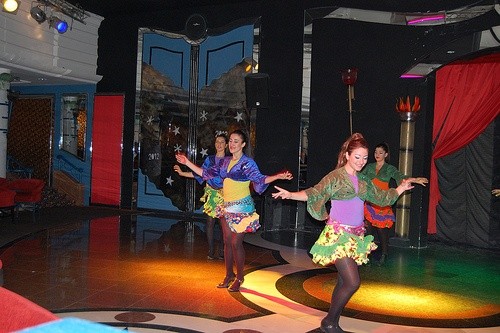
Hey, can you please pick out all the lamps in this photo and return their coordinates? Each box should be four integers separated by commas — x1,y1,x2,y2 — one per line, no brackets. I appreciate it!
241,60,251,72
2,0,68,34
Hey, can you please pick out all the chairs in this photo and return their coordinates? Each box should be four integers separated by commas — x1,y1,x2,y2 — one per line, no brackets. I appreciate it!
0,177,47,217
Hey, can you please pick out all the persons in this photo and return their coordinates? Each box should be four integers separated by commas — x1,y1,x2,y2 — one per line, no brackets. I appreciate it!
269,130,415,333
348,141,430,264
174,133,230,259
491,188,500,197
175,129,293,292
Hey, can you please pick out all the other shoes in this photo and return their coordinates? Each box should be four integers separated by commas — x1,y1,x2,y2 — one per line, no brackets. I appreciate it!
219,247,224,260
364,254,375,266
377,254,387,266
207,248,216,259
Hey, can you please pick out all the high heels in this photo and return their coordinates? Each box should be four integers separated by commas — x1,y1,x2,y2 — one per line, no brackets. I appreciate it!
228,278,244,292
320,317,344,333
217,272,236,288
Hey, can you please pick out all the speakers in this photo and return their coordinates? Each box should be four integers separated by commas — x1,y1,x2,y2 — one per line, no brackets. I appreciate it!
246,74,272,109
